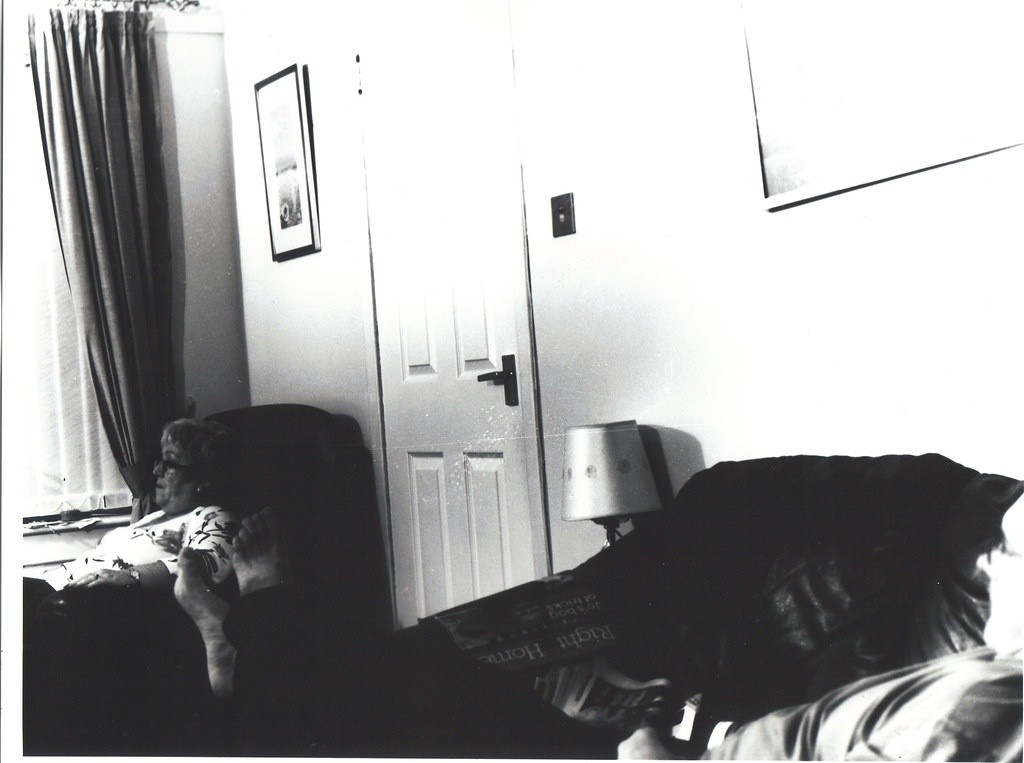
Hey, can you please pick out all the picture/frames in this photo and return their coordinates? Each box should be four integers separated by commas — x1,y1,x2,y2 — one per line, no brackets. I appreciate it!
252,62,323,264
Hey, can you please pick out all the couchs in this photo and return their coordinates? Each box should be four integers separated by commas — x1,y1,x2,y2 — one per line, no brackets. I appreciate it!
22,402,386,760
389,453,1022,762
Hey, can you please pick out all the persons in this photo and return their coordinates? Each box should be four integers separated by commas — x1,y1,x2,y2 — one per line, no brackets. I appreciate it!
24,419,237,625
175,485,1024,763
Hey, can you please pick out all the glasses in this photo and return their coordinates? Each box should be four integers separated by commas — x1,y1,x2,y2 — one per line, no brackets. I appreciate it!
987,544,1024,564
153,458,195,474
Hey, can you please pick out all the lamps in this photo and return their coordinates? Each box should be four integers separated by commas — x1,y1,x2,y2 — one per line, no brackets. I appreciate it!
561,420,664,554
